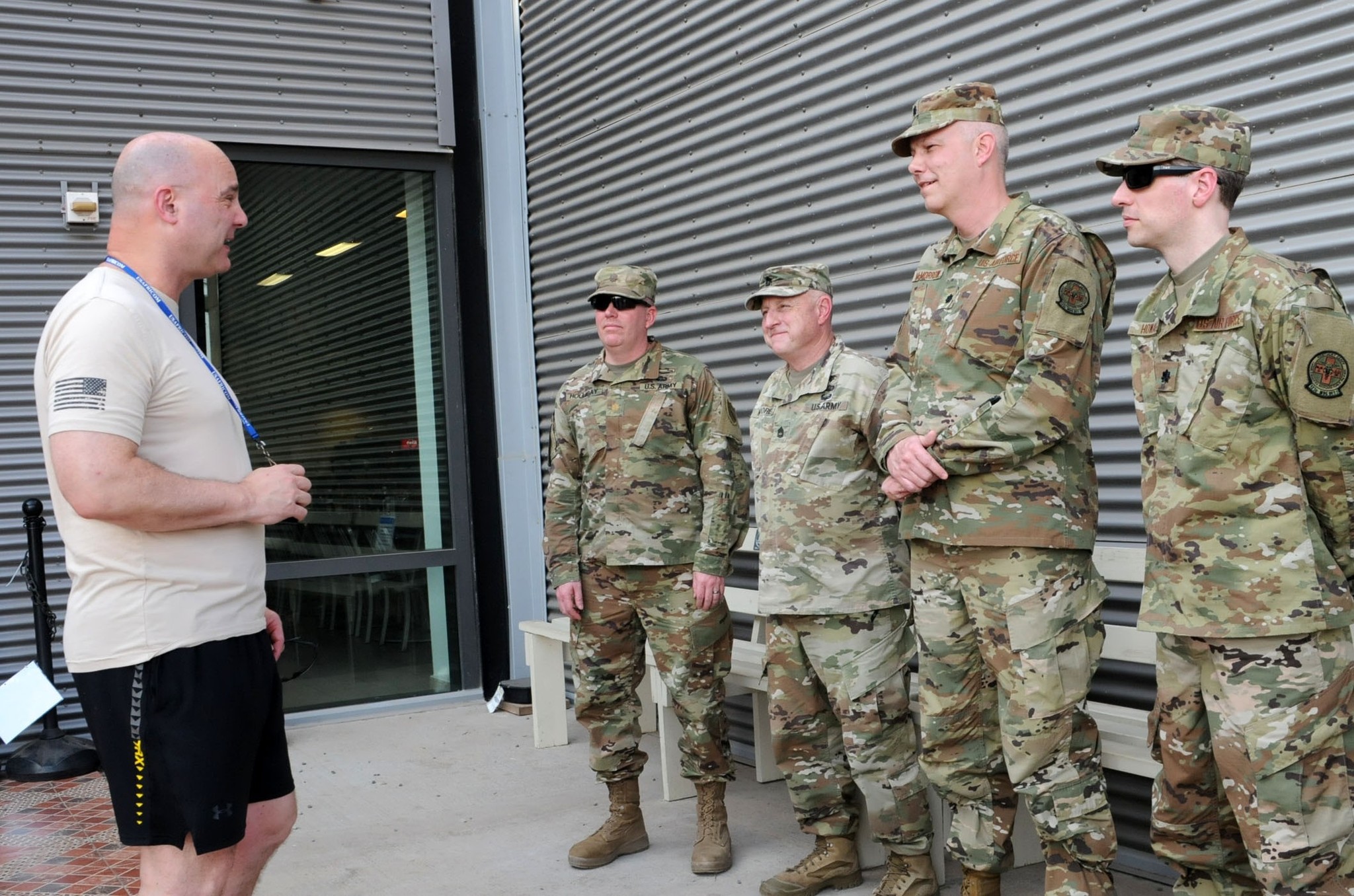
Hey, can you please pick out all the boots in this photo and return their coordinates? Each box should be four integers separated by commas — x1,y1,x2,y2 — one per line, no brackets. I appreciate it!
567,776,649,869
690,781,732,873
959,865,1001,896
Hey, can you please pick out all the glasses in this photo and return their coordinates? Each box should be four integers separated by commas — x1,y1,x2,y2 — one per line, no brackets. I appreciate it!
1122,165,1224,189
591,294,650,311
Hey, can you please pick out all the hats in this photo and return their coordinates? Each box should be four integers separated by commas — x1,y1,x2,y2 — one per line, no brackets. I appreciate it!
1095,103,1252,177
891,82,1005,157
587,265,658,302
745,263,834,310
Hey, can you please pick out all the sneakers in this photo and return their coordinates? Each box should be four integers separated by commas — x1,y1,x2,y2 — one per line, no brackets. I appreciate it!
759,835,862,896
873,853,940,896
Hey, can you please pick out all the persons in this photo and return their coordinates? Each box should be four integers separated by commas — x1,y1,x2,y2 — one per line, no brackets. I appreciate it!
34,132,311,896
544,264,748,874
864,82,1117,896
747,265,940,896
1097,106,1354,896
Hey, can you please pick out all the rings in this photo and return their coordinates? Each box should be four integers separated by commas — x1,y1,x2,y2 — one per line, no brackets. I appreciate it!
712,591,720,594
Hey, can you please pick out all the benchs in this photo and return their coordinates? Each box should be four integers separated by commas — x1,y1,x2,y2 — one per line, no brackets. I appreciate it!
519,524,788,802
907,543,1182,888
269,496,426,658
